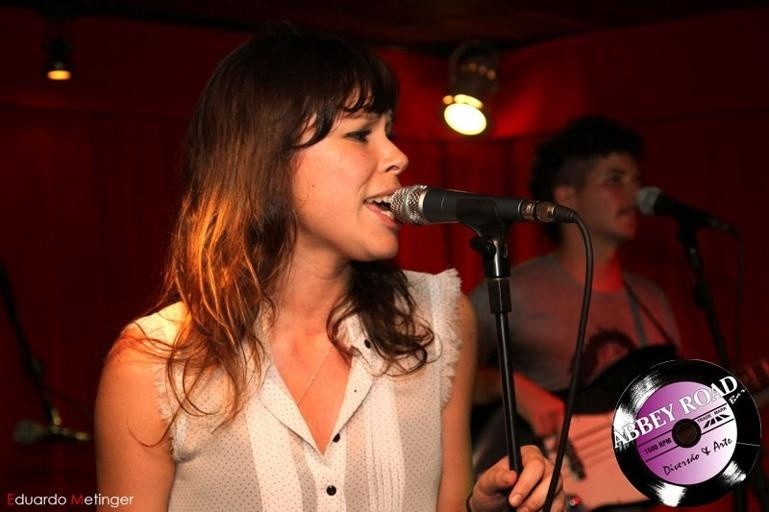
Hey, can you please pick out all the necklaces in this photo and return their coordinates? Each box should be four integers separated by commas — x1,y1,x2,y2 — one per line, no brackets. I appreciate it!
295,343,335,408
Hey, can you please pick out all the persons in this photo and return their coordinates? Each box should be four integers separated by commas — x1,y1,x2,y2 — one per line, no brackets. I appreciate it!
92,24,582,512
465,112,685,437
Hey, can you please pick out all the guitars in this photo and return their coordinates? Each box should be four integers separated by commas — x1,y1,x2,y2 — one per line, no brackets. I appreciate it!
471,341,768,511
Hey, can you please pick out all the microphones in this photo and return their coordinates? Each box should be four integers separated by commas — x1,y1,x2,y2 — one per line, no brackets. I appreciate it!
390,185,556,225
635,187,738,236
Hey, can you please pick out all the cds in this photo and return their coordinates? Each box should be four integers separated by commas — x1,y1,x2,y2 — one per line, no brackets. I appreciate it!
610,356,762,510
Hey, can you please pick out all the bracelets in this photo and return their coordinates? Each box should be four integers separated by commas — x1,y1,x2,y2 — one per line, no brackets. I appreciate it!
466,494,471,511
